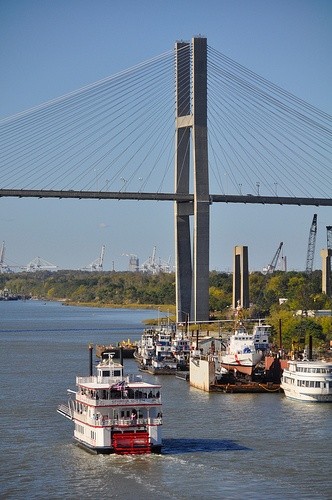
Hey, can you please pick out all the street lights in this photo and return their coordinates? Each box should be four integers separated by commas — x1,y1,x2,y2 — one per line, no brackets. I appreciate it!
238,183,243,196
139,178,143,193
178,309,189,340
255,181,261,196
273,182,278,196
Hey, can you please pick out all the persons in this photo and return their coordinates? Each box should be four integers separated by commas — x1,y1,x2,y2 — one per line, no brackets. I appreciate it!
129,411,137,422
91,387,161,400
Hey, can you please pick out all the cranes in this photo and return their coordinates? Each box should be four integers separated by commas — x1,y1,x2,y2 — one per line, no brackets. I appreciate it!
140,245,175,273
89,245,106,271
0,240,14,274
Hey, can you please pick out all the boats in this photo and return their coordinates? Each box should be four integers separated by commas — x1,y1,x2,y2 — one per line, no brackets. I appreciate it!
95,319,271,382
280,349,332,403
55,343,164,455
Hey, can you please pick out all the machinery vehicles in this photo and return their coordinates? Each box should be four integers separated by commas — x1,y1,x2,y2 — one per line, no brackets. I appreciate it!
301,214,318,318
255,241,284,306
